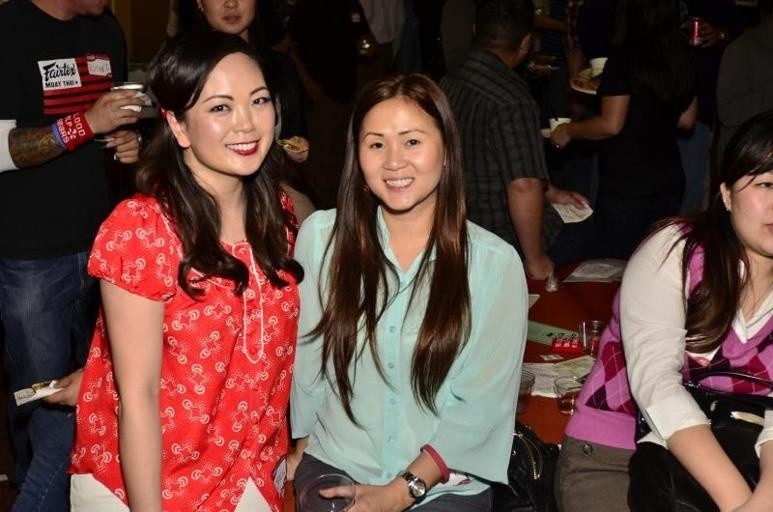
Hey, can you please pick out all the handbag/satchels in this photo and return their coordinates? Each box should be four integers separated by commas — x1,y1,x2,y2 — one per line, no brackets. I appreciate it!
491,419,560,511
626,383,773,512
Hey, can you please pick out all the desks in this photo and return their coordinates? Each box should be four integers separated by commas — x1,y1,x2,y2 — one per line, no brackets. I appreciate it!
512,259,623,445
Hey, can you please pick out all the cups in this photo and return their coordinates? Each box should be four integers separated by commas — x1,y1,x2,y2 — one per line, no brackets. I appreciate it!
549,117,572,134
579,318,604,353
589,56,606,74
298,470,357,511
108,84,145,116
552,373,588,415
514,369,536,417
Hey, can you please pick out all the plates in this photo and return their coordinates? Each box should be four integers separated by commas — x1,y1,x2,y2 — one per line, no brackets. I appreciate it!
578,67,591,80
539,128,552,139
567,77,597,96
